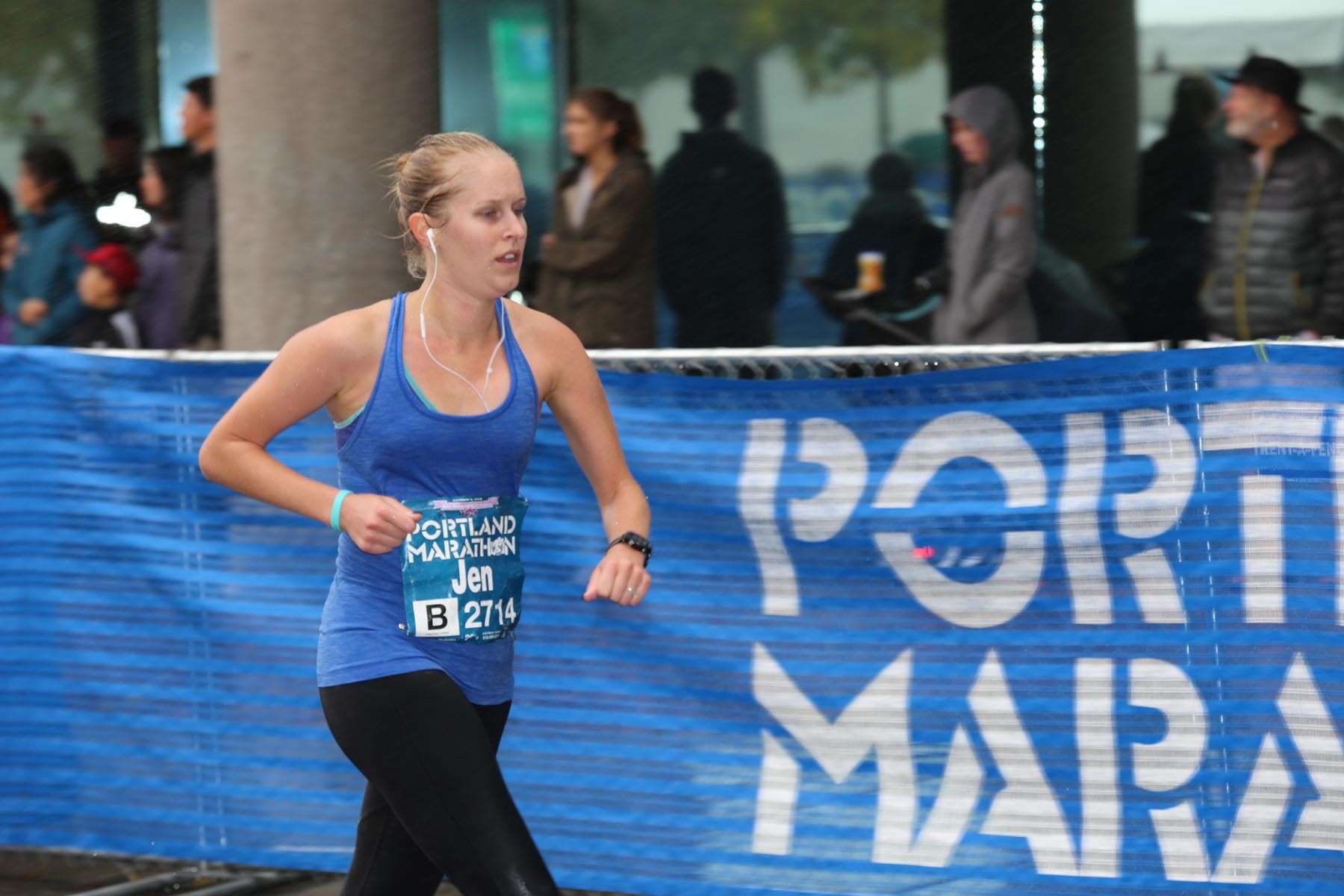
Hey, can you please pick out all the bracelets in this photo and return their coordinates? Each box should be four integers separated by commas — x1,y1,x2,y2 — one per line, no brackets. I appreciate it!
329,488,355,532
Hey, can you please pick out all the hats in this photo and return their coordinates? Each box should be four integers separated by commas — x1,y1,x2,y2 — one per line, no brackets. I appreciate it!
76,243,138,292
1212,53,1319,116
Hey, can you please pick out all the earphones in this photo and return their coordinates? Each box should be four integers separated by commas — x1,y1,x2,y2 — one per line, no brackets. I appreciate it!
426,227,438,253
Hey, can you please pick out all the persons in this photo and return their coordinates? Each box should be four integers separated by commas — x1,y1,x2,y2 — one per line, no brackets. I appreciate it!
501,50,1344,350
1,76,216,352
199,133,653,896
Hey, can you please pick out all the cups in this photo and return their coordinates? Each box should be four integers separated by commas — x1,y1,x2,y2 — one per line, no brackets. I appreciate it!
858,254,884,292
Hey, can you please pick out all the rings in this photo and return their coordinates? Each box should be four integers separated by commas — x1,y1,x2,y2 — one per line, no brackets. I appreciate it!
626,585,635,593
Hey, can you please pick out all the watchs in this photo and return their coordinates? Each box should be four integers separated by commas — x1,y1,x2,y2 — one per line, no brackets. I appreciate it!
602,531,651,567
